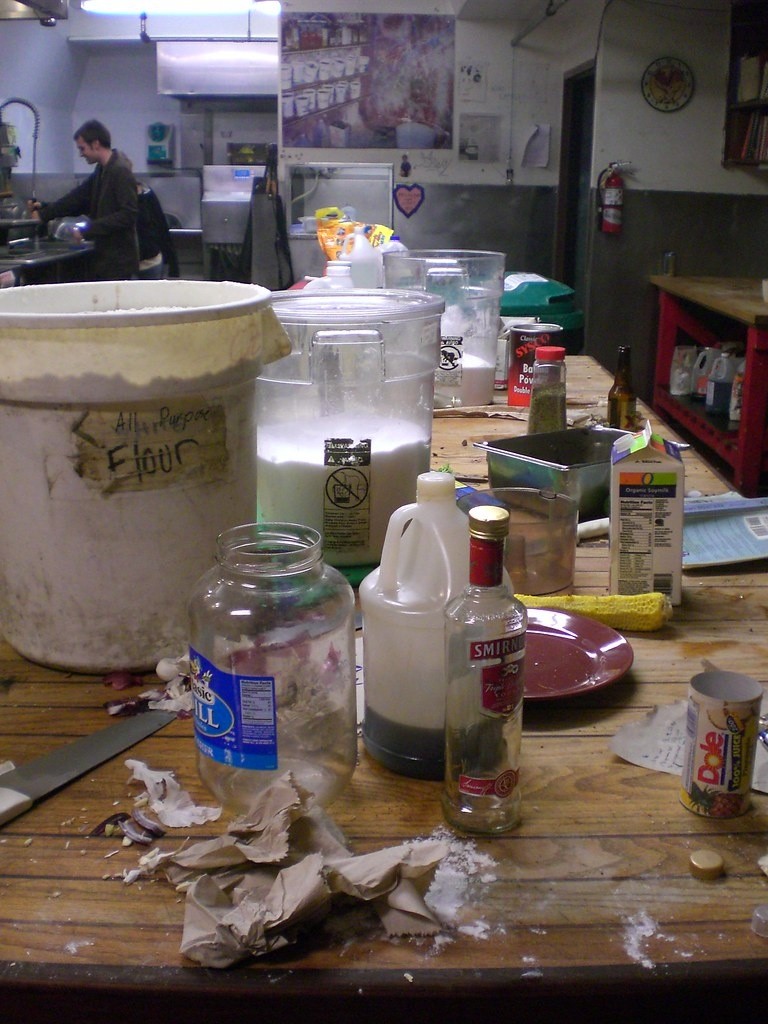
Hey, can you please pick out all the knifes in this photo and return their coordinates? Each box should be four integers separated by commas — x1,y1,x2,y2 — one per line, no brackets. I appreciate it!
0,710,177,826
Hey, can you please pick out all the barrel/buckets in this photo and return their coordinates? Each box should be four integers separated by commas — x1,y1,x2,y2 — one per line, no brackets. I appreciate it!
258,290,446,564
0,281,290,677
383,249,507,410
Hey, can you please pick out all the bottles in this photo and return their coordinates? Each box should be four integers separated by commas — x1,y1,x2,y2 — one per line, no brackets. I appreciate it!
608,346,637,433
360,471,512,782
189,523,357,815
528,346,568,435
706,352,736,418
692,347,714,406
444,505,528,834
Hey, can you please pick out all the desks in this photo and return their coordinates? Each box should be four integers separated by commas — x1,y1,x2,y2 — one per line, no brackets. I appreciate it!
0,355,768,990
649,272,768,494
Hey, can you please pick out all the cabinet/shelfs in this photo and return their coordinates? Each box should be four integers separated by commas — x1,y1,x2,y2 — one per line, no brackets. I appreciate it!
721,0,767,167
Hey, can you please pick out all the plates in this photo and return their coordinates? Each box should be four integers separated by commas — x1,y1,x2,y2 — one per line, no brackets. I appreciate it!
524,607,635,701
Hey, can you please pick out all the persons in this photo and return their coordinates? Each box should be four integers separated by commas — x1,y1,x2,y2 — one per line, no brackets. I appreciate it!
29,119,139,281
112,148,179,279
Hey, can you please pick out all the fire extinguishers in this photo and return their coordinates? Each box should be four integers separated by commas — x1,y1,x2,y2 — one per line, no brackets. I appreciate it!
596,159,633,235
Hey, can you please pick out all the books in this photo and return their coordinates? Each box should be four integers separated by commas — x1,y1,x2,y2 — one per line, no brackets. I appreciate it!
682,491,768,569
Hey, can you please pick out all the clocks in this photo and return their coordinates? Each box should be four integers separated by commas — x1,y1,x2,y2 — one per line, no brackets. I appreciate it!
641,57,693,111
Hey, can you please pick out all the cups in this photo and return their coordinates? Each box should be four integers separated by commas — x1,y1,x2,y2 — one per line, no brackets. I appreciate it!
509,323,563,406
458,487,578,599
677,673,765,818
